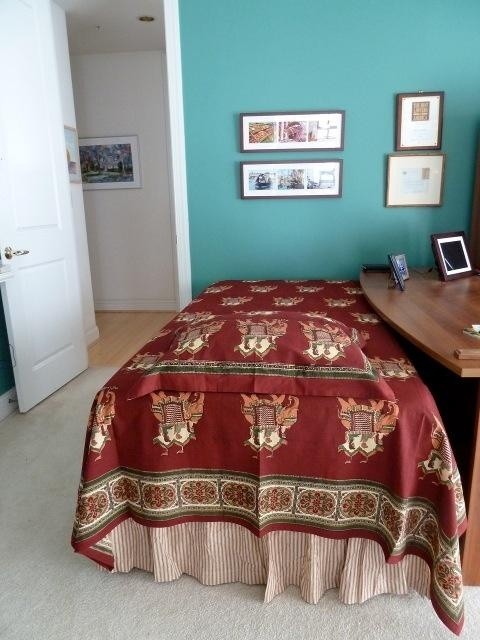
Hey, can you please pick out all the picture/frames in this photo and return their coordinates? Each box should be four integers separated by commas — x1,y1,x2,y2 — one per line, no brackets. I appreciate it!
388,230,478,291
386,90,447,209
239,109,344,201
61,124,143,193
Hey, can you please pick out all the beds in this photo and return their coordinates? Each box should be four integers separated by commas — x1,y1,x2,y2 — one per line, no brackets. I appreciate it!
97,278,453,596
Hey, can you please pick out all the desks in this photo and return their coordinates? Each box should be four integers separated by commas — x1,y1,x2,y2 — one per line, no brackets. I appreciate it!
356,266,478,593
